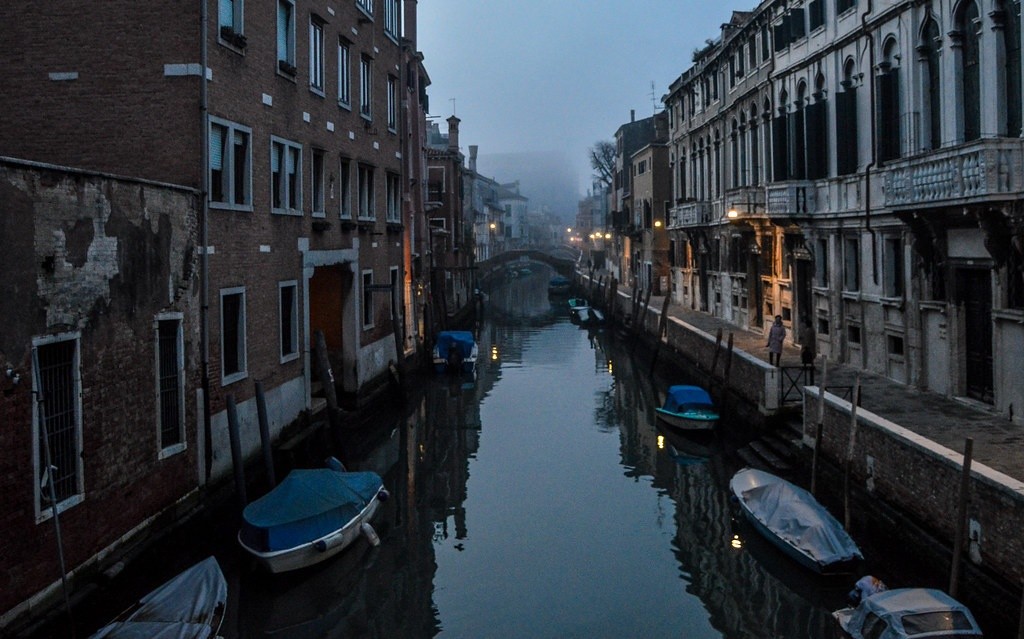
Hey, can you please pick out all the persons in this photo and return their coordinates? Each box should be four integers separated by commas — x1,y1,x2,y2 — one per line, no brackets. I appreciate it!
799,320,815,370
765,315,786,367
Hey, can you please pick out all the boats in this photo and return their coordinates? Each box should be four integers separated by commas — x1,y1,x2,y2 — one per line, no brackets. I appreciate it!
569,297,591,319
432,331,478,373
830,574,984,639
237,468,390,576
654,384,720,429
83,555,229,639
729,467,864,572
